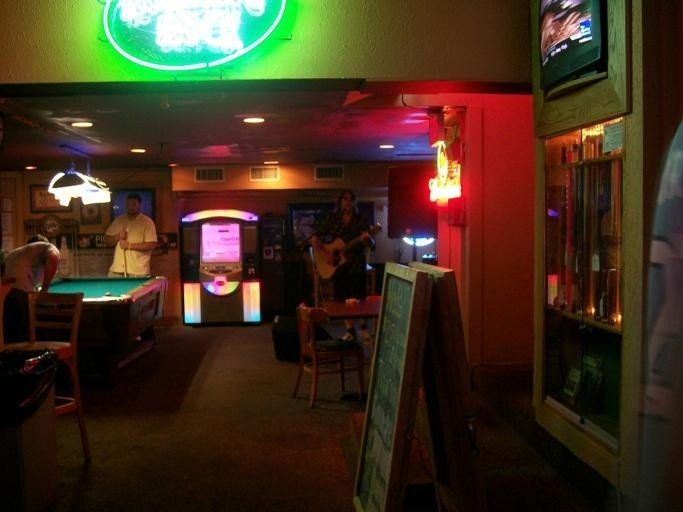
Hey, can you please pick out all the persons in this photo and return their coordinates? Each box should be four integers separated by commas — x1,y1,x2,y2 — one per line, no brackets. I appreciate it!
298,188,376,345
105,193,160,277
542,11,586,52
0,234,59,342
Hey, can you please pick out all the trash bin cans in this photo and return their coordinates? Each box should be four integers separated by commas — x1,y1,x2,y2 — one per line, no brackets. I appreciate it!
0,348,58,512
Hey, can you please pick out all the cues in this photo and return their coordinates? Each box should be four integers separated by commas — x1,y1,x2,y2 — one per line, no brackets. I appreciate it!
121,223,127,277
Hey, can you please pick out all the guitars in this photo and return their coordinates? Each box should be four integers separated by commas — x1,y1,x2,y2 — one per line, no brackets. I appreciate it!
316,222,382,280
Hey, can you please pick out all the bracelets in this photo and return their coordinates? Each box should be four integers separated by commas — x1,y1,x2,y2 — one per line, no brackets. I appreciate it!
128,243,133,250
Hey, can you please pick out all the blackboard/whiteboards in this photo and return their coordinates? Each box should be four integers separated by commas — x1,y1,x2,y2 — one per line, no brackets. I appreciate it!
352,263,428,512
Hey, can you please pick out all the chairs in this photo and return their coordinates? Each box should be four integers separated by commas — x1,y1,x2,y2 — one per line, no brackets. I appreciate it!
292,302,365,408
5,292,93,465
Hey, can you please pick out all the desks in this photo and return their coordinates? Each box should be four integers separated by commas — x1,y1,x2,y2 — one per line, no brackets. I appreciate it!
328,300,381,375
33,276,168,358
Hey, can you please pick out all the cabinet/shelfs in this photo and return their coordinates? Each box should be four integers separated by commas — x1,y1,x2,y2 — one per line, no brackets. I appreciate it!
532,115,643,486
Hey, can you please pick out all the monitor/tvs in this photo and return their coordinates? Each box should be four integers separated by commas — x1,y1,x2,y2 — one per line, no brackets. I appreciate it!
536,0,608,90
109,187,156,225
